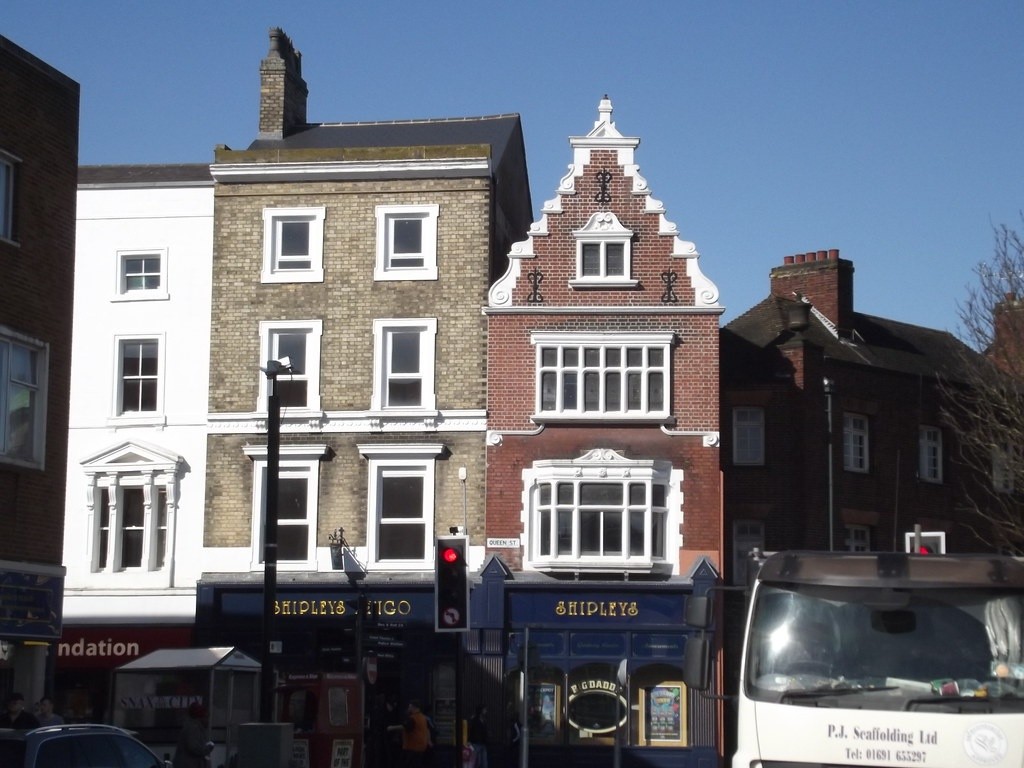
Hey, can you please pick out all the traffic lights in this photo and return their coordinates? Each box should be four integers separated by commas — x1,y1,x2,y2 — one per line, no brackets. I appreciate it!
436,537,471,636
905,531,946,558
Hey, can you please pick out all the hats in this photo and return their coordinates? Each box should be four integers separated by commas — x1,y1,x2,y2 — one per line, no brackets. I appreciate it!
190,703,208,718
6,693,24,701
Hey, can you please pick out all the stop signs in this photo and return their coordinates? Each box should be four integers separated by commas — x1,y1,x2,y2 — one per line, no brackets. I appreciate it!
365,655,376,683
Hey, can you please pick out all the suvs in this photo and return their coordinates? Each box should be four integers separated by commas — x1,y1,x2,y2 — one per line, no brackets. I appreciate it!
0,724,163,768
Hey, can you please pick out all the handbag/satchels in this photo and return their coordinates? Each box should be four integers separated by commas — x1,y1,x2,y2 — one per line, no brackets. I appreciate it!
462,745,476,768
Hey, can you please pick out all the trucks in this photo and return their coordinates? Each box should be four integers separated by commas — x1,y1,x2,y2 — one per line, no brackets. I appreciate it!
684,549,1024,768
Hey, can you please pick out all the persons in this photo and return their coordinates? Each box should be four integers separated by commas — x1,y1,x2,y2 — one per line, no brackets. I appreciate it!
468,704,489,768
172,703,216,768
374,698,438,768
33,696,66,727
0,692,41,729
